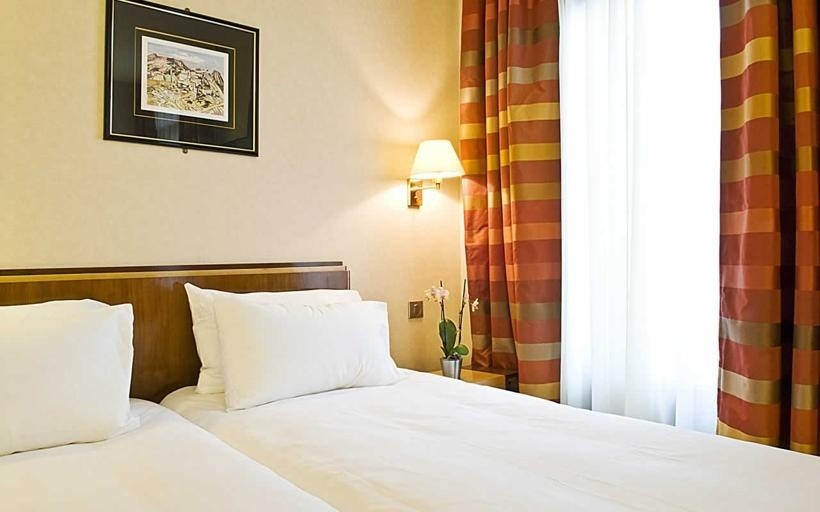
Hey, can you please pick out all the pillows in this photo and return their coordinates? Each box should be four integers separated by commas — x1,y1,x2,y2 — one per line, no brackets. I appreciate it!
0,299,136,456
212,293,400,412
181,282,363,393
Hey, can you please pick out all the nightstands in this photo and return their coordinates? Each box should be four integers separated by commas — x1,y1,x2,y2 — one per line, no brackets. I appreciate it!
430,368,506,389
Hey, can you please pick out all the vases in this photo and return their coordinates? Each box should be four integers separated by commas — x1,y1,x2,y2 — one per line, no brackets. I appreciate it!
441,357,461,381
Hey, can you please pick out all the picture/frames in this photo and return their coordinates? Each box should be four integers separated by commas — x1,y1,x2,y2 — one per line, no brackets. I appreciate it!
102,0,260,156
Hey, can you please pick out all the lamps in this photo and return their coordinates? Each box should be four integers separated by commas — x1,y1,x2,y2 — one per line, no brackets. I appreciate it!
405,139,468,208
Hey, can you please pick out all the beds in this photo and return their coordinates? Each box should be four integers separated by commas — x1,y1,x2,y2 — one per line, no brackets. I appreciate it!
0,398,342,512
161,362,819,512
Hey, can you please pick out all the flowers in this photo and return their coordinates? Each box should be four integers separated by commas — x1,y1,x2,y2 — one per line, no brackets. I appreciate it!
424,280,480,361
